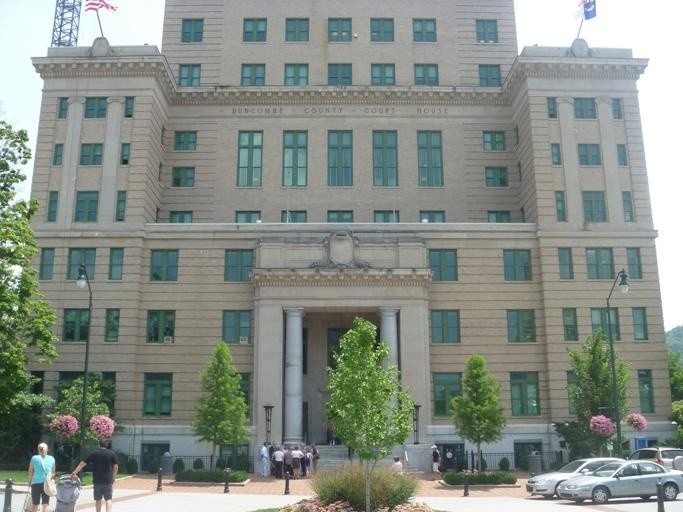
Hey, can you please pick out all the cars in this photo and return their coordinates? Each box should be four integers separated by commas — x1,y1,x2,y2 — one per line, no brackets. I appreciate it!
525,457,652,499
555,460,682,504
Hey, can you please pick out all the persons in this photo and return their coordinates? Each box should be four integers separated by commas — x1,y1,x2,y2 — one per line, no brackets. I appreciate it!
70,437,119,512
258,441,321,480
430,445,443,480
27,440,56,512
326,416,336,446
391,456,402,472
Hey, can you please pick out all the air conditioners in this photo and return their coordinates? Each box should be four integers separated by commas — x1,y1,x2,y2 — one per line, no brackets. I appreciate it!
164,336,172,344
239,336,248,345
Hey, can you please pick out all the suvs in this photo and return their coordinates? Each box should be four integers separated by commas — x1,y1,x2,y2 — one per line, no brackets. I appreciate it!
624,446,682,473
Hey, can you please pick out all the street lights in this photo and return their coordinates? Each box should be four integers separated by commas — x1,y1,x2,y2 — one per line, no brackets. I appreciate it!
261,402,274,447
76,260,93,480
605,267,629,456
412,401,422,444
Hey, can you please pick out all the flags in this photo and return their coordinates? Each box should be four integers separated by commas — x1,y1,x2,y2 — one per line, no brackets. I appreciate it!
576,0,597,21
84,0,119,14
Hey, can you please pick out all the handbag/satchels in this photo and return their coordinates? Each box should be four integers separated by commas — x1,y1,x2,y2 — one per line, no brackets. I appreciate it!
43,473,57,497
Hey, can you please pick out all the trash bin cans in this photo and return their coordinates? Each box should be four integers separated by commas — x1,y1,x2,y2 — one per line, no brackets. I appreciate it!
528,450,541,476
160,451,173,474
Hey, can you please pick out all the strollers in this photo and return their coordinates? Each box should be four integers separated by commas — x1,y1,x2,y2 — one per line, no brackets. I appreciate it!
20,482,43,512
54,473,81,512
